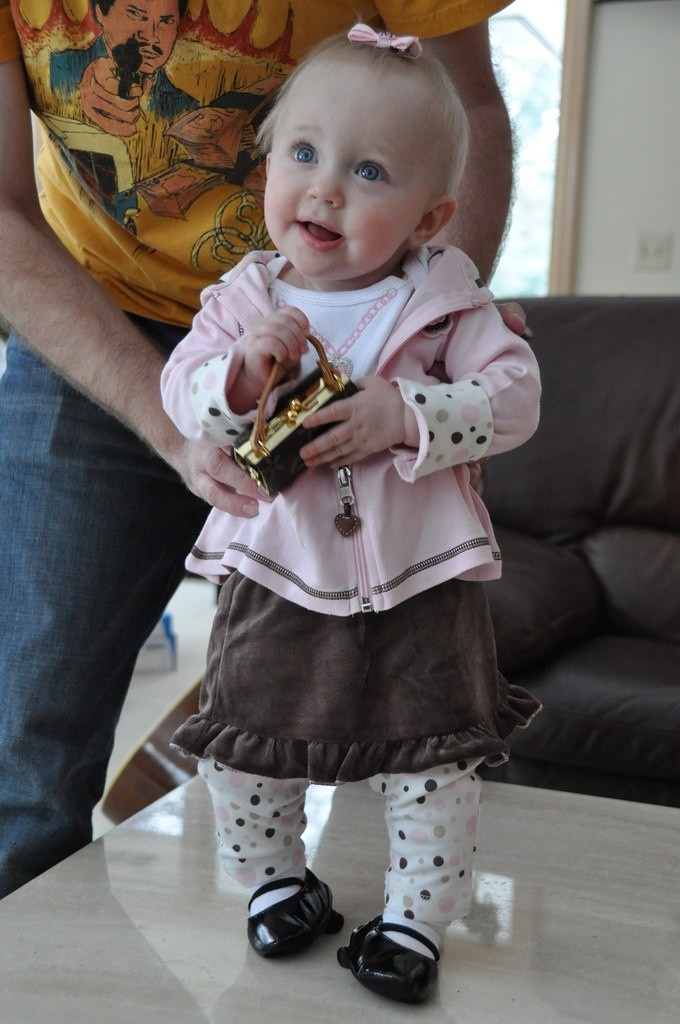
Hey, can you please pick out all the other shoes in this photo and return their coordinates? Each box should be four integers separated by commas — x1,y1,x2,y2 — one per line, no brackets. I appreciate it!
247,867,344,957
337,914,440,1004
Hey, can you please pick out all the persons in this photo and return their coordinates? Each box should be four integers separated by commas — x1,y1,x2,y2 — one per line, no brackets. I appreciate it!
160,22,544,1005
0,0,517,900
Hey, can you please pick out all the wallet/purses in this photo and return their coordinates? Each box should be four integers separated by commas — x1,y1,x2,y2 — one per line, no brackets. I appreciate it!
231,334,360,498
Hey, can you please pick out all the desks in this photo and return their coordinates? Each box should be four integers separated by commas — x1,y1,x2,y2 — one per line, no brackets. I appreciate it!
1,771,678,1024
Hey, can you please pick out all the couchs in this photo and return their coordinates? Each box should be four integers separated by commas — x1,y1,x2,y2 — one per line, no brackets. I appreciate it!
473,294,679,807
